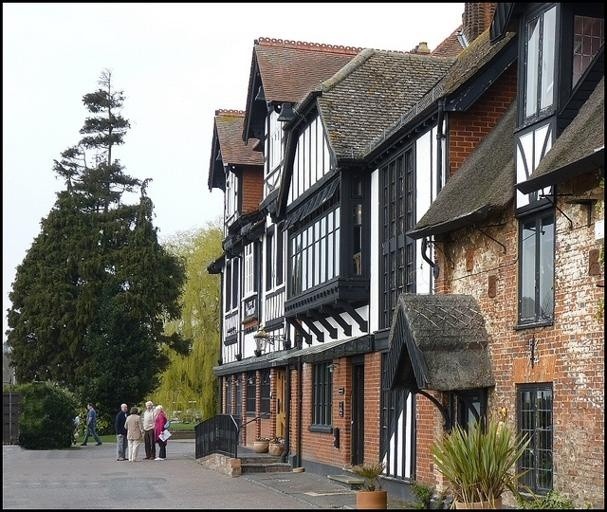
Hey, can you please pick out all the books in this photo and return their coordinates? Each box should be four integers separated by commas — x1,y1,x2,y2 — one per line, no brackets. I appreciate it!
158,429,172,442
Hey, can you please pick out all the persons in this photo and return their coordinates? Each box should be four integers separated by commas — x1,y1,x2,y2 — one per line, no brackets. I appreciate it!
141,401,160,461
122,407,144,462
78,403,103,446
152,405,169,461
113,403,129,461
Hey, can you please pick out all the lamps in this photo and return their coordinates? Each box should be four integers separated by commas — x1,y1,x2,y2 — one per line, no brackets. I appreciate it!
225,374,272,386
328,362,340,372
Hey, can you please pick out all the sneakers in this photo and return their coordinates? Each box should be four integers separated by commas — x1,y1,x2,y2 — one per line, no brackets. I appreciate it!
116,457,128,461
95,442,102,446
144,457,167,460
80,443,88,446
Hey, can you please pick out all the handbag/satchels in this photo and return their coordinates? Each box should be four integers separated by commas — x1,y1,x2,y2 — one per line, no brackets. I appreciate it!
159,420,173,442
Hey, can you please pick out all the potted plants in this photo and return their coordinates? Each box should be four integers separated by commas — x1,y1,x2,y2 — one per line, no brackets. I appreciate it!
268,436,284,456
253,435,270,452
409,482,447,510
427,416,541,509
352,463,387,509
